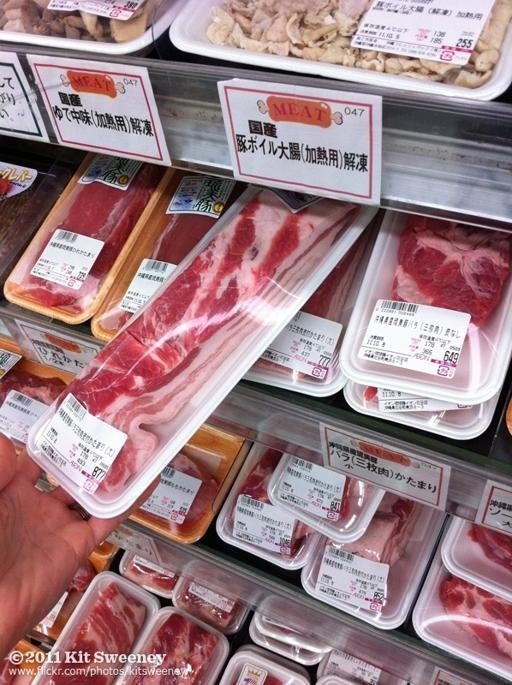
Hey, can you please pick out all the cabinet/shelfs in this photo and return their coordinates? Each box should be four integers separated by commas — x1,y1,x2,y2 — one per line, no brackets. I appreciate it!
0,1,512,685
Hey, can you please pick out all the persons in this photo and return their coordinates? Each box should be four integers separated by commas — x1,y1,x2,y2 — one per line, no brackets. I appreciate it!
0,436,160,661
